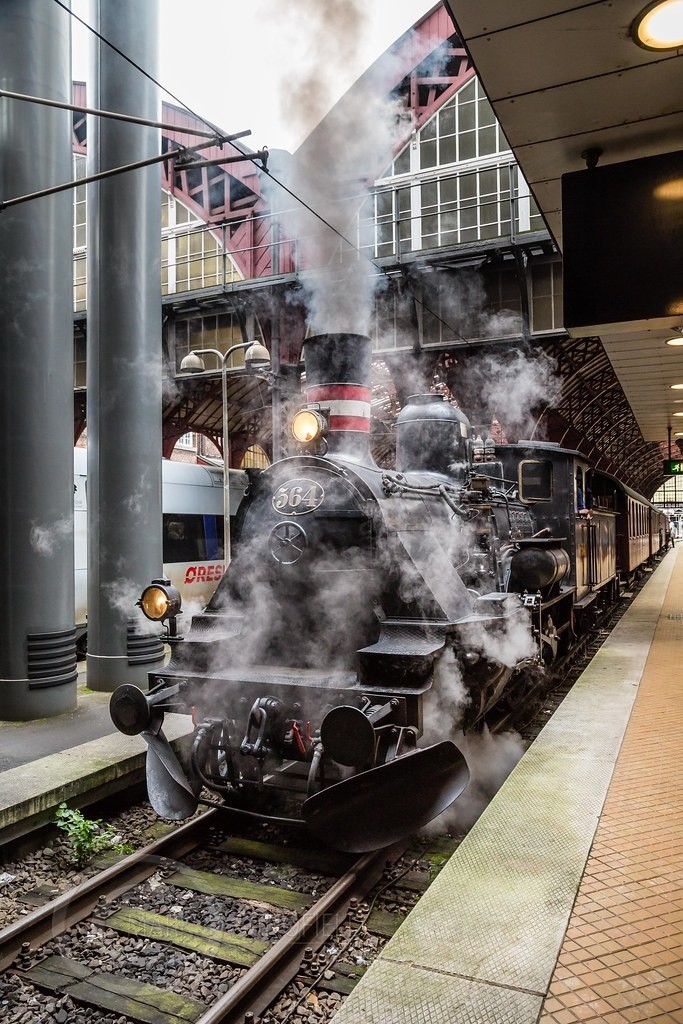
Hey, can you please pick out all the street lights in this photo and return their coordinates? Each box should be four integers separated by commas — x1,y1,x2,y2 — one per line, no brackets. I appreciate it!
177,337,278,574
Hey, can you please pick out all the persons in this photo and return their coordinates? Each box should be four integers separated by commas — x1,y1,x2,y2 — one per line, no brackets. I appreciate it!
659,522,676,551
577,474,594,520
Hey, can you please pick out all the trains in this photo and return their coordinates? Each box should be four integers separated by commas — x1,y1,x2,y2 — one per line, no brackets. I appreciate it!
64,447,275,648
102,334,674,858
